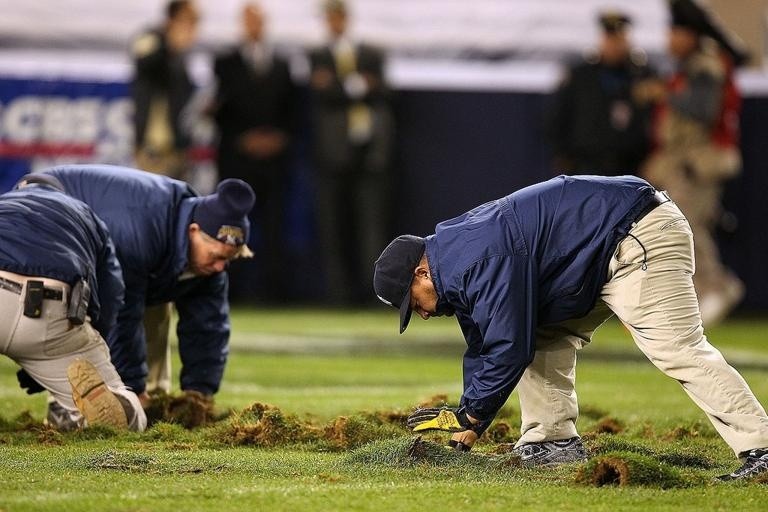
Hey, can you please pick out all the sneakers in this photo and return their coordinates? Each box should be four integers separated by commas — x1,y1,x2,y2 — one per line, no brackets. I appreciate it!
43,357,130,436
713,448,768,483
511,438,588,467
698,274,744,327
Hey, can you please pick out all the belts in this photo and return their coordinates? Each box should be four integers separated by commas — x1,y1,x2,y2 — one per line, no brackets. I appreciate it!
617,189,671,243
0,278,72,302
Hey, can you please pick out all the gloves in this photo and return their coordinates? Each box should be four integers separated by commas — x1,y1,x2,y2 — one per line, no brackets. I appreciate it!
408,405,474,432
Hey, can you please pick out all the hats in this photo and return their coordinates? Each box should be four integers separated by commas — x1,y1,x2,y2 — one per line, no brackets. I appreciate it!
191,177,257,247
372,232,428,335
597,11,632,32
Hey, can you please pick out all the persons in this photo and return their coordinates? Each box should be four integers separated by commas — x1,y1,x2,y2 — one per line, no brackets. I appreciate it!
214,1,311,303
634,0,751,326
0,174,148,434
299,0,391,308
374,174,768,481
131,0,219,186
542,12,665,183
13,165,255,426
